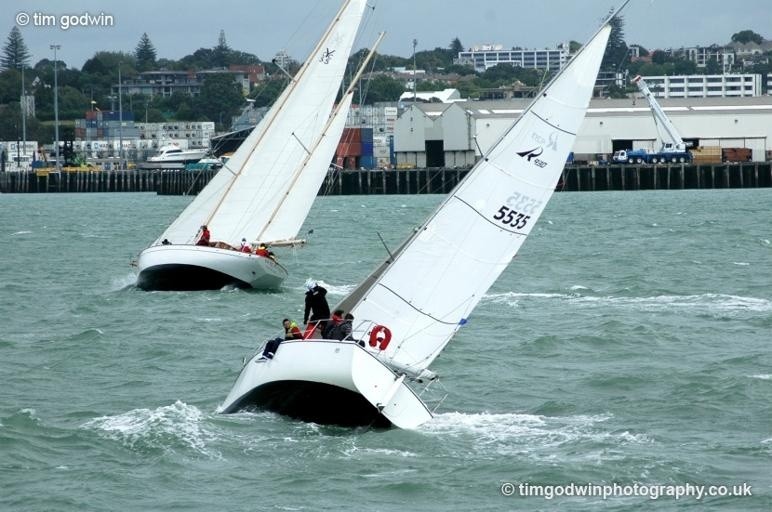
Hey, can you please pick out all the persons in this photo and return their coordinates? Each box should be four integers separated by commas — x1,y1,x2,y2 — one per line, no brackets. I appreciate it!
256,243,270,258
196,225,210,246
161,239,171,245
320,310,344,339
327,312,365,348
238,238,253,253
255,319,304,363
304,281,330,327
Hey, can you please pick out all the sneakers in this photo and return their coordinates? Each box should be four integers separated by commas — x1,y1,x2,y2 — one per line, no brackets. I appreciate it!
256,358,266,362
263,354,273,360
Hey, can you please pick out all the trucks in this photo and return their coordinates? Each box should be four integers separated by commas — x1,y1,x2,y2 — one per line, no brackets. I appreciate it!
614,148,647,164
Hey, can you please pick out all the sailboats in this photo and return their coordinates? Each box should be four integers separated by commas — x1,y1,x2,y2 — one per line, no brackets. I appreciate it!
132,1,388,290
216,2,628,430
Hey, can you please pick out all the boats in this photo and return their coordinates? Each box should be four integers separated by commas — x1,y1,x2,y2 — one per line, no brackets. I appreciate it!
147,148,203,164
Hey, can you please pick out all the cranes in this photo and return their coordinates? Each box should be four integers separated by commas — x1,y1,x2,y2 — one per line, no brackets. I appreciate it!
631,74,687,165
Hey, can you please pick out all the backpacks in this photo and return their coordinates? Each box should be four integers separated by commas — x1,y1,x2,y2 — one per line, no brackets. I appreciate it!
321,320,347,339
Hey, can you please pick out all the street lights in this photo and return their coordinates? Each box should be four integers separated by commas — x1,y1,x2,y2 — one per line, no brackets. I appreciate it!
50,45,61,169
